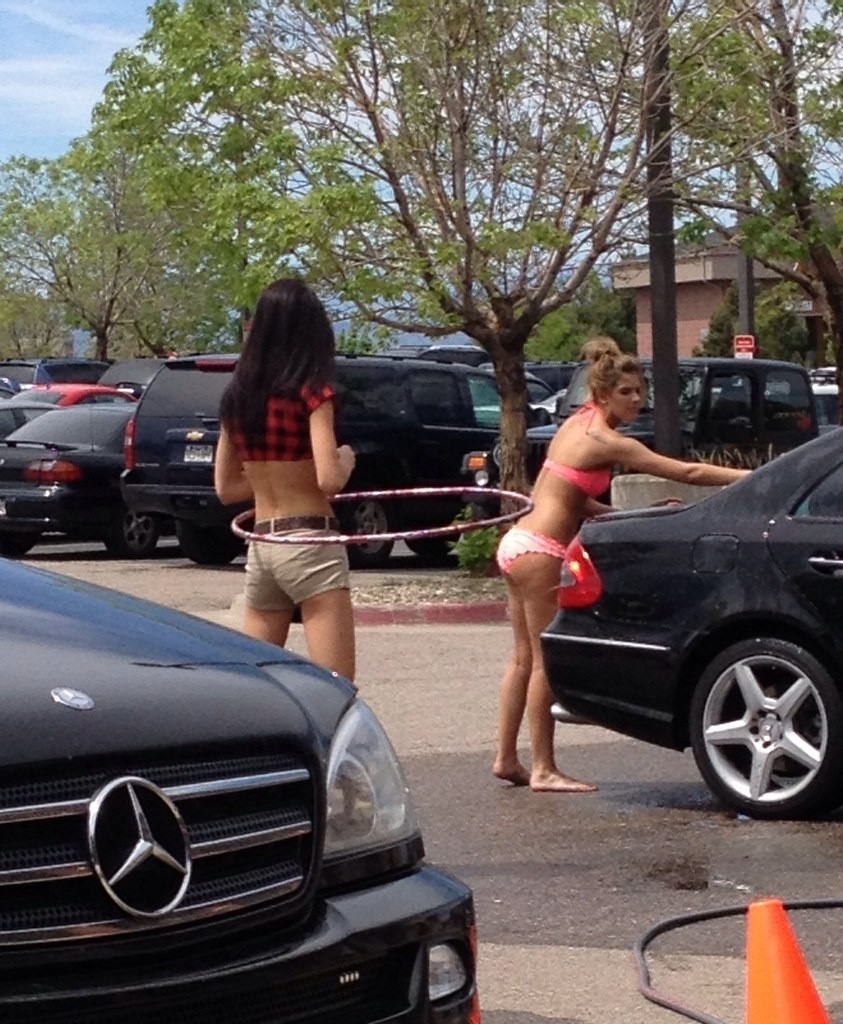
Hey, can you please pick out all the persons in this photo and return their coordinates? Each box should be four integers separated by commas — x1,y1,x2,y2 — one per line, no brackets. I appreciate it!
209,280,359,682
492,335,753,791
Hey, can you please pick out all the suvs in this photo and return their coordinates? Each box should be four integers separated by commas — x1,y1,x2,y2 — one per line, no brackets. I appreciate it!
116,354,554,570
1,343,843,567
0,551,482,1023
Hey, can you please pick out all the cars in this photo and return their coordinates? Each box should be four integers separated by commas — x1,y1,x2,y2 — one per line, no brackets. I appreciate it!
533,423,842,821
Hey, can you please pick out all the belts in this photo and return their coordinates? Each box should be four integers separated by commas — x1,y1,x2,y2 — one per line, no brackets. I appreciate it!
254,517,343,537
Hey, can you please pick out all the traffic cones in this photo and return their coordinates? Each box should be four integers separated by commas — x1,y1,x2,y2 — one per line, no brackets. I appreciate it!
742,898,831,1024
